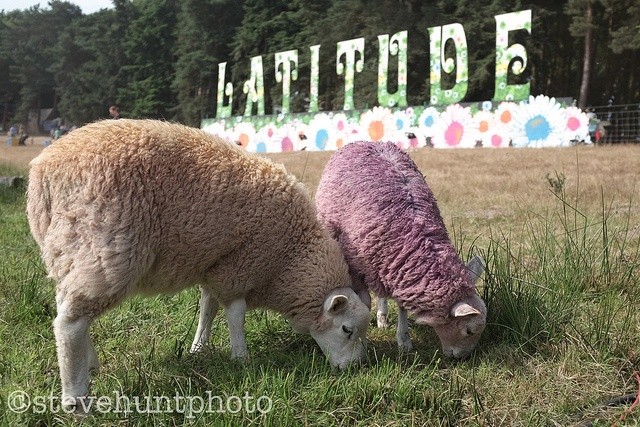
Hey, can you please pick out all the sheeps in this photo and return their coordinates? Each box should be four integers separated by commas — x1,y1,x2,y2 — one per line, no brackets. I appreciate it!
25,118,371,416
314,140,488,361
